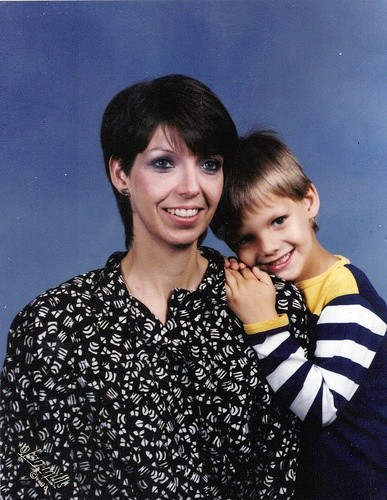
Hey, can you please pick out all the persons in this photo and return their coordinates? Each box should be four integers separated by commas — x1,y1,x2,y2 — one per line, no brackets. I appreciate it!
0,73,308,500
209,129,387,500
